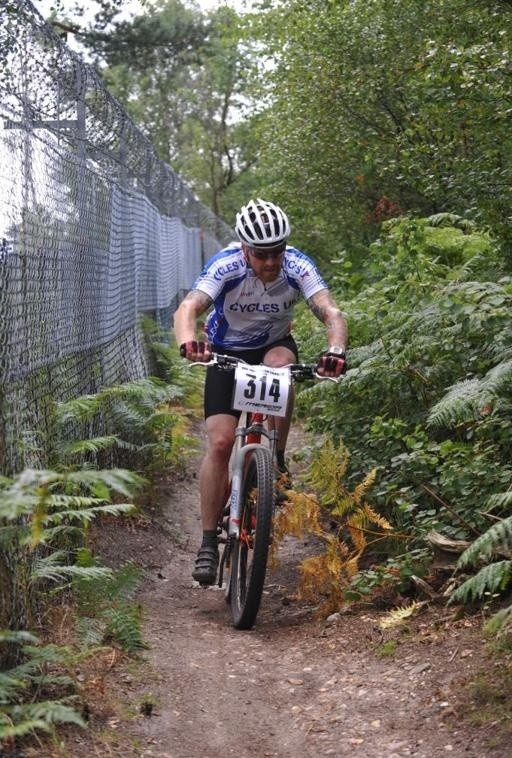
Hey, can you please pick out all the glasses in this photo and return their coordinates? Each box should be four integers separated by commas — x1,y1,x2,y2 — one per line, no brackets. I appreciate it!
249,243,286,260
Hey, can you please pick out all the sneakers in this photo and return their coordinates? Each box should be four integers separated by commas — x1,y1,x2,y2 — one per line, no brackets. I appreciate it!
277,465,291,489
191,546,219,583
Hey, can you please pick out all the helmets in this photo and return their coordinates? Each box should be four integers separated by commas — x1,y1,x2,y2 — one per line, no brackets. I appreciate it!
234,196,291,249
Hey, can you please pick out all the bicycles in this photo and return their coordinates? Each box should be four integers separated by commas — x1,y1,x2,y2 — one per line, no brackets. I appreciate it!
180,349,348,628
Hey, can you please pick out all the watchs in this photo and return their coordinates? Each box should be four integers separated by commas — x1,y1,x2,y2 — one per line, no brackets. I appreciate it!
326,346,346,356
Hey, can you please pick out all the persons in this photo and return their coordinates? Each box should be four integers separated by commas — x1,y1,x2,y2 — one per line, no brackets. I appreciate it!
173,198,348,584
